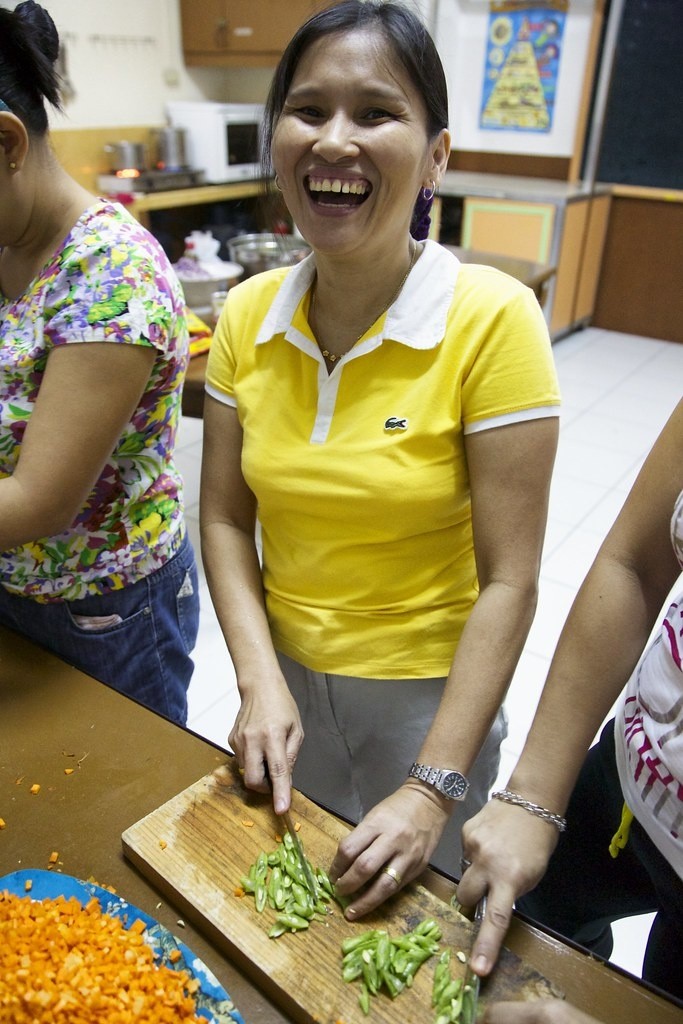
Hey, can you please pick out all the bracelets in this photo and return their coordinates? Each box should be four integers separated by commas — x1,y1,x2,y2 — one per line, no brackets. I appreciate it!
490,786,568,836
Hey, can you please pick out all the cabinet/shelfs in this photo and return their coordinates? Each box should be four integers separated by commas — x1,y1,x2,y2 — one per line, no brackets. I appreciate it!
179,0,346,67
428,196,610,345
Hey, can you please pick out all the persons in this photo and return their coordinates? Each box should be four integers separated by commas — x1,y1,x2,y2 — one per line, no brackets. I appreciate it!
457,398,683,998
200,6,565,920
0,1,202,728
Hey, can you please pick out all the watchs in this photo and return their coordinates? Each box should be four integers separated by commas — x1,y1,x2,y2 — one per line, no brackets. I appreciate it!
405,763,475,807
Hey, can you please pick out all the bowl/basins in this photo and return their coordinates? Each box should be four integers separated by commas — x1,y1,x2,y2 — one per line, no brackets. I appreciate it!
226,233,312,284
171,260,246,306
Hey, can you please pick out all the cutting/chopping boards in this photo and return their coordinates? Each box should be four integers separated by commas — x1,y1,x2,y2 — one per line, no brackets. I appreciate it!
120,750,683,1024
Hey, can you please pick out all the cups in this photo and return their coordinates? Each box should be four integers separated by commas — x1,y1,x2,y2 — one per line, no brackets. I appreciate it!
150,127,188,171
104,141,144,174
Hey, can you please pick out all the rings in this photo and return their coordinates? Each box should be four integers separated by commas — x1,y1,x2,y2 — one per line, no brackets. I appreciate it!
381,864,403,888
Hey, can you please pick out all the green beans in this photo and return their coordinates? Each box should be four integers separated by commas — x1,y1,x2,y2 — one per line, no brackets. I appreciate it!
242,833,466,1024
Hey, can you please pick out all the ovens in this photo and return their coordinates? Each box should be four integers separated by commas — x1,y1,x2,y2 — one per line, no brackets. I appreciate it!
164,100,278,185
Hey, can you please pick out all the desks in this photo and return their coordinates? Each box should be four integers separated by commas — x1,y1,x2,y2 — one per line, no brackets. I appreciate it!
0,624,683,1024
128,179,285,234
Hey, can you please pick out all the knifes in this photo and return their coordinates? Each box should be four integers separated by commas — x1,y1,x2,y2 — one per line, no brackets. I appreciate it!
460,893,488,1024
263,758,318,902
